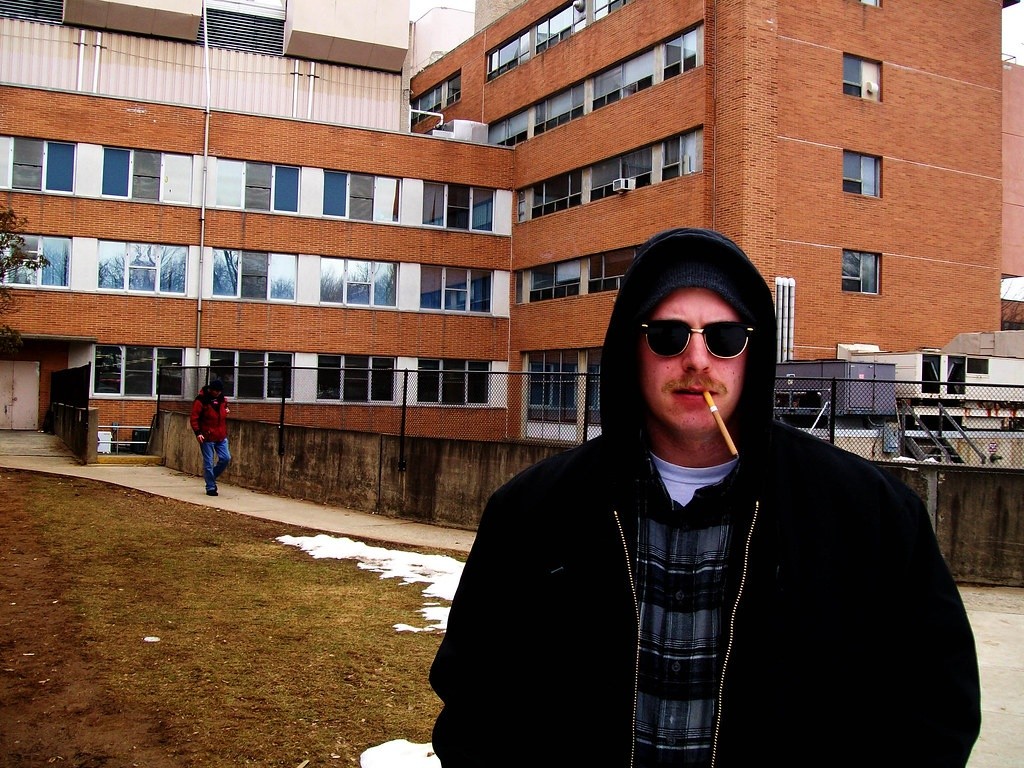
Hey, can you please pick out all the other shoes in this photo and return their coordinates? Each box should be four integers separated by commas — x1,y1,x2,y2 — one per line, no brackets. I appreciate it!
207,490,218,496
215,485,217,492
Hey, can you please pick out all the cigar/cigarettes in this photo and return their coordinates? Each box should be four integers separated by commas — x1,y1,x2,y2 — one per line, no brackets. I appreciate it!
703,392,737,455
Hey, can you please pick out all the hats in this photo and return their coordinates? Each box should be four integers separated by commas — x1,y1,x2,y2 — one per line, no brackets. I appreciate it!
633,254,761,349
209,379,224,391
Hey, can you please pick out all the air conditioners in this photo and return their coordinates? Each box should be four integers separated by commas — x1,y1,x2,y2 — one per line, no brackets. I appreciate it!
612,178,635,194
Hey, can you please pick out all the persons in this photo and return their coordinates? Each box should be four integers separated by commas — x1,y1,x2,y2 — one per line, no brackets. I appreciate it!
430,227,981,768
190,380,231,496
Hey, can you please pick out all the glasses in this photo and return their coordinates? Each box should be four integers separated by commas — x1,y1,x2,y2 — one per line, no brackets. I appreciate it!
639,319,754,357
